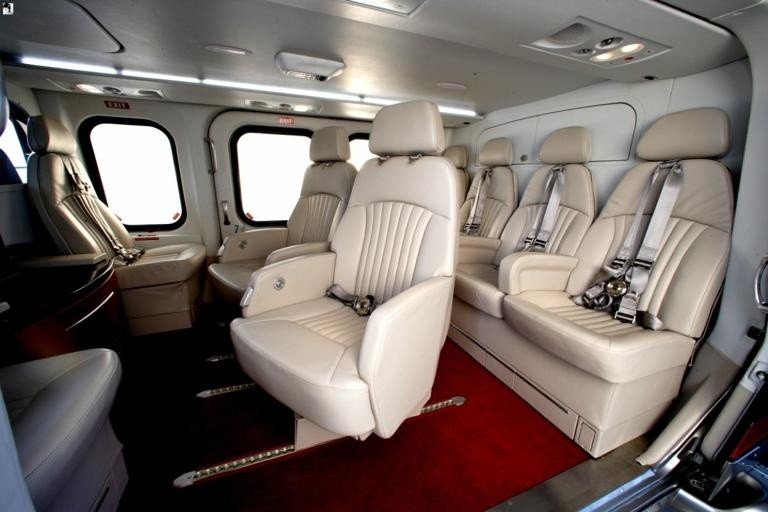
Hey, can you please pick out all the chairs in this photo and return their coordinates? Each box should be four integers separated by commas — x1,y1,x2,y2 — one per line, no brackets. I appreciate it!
442,145,470,210
502,108,733,383
455,126,598,319
25,113,207,337
0,344,130,512
453,137,518,265
229,99,459,441
207,125,358,299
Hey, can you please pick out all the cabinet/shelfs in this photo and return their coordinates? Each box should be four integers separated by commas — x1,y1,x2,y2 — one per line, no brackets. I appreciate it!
12,253,128,375
